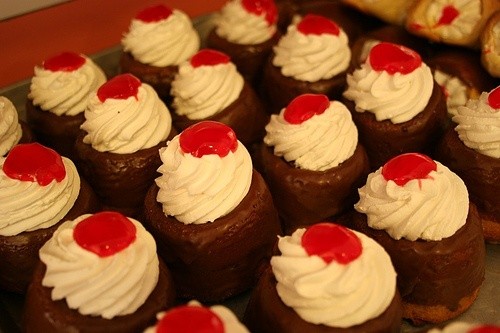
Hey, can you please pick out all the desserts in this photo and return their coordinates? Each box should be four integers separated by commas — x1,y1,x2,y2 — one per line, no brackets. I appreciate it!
0,0,500,333
